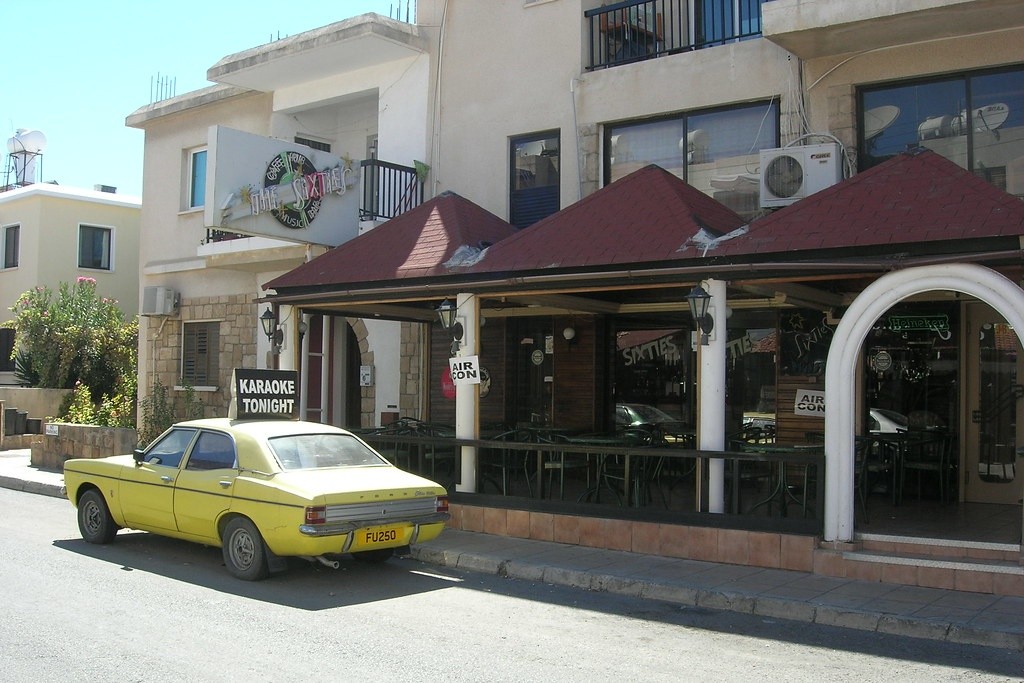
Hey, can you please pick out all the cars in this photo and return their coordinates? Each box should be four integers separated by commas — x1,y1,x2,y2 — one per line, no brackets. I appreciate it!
867,407,943,453
64,416,452,585
650,411,776,445
615,402,688,441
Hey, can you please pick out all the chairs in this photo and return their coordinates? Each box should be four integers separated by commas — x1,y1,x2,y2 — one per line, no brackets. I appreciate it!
346,417,457,496
477,416,591,506
804,424,960,528
635,419,776,528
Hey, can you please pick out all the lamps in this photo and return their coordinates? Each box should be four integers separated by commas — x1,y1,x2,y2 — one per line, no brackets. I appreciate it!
298,321,307,343
260,306,283,346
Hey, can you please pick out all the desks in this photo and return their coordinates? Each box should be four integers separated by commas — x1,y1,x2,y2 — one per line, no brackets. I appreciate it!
740,442,818,520
566,433,646,510
438,429,501,491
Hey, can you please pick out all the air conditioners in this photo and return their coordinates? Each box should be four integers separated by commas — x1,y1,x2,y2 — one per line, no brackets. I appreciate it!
142,285,177,318
757,140,847,210
